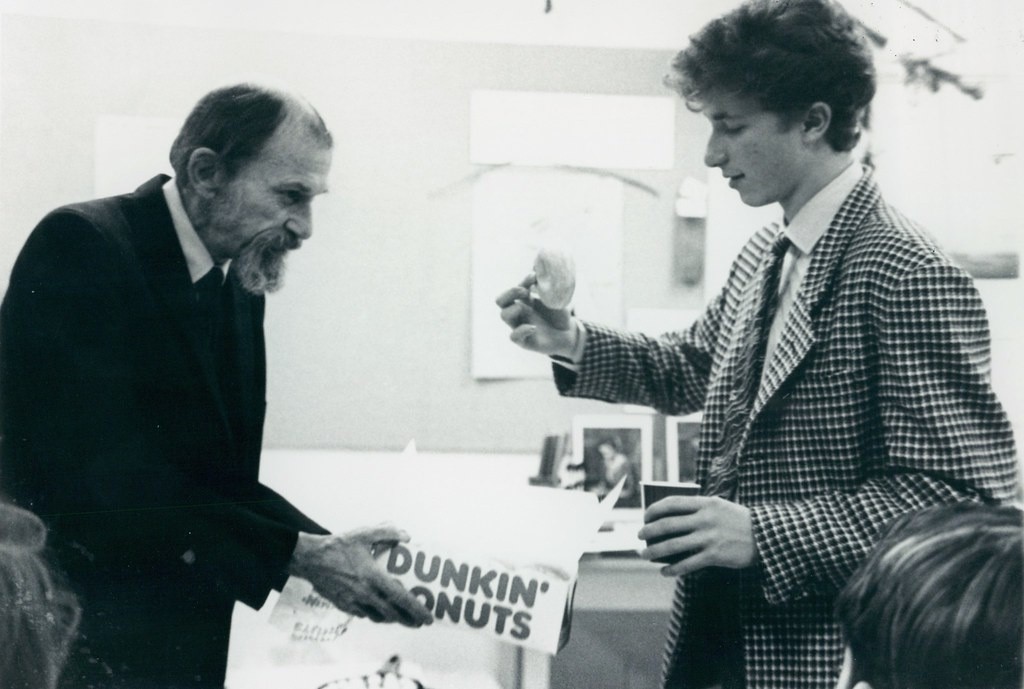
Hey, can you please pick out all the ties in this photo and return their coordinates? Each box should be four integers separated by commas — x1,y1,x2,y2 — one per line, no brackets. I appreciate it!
703,231,792,498
194,266,227,312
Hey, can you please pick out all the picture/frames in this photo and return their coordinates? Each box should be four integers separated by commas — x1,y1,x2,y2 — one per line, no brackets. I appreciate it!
664,413,703,485
572,415,655,528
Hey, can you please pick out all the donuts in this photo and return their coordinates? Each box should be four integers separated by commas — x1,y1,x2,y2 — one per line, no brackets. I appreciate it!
532,243,575,309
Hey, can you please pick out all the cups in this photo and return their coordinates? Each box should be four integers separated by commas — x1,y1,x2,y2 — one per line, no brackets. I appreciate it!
639,482,703,564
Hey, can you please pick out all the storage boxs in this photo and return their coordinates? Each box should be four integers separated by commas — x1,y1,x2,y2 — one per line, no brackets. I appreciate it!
358,439,627,656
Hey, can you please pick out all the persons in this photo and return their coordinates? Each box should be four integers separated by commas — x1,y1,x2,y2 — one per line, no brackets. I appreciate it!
0,83,435,689
833,505,1024,689
590,434,636,506
1,547,62,689
497,1,1018,689
